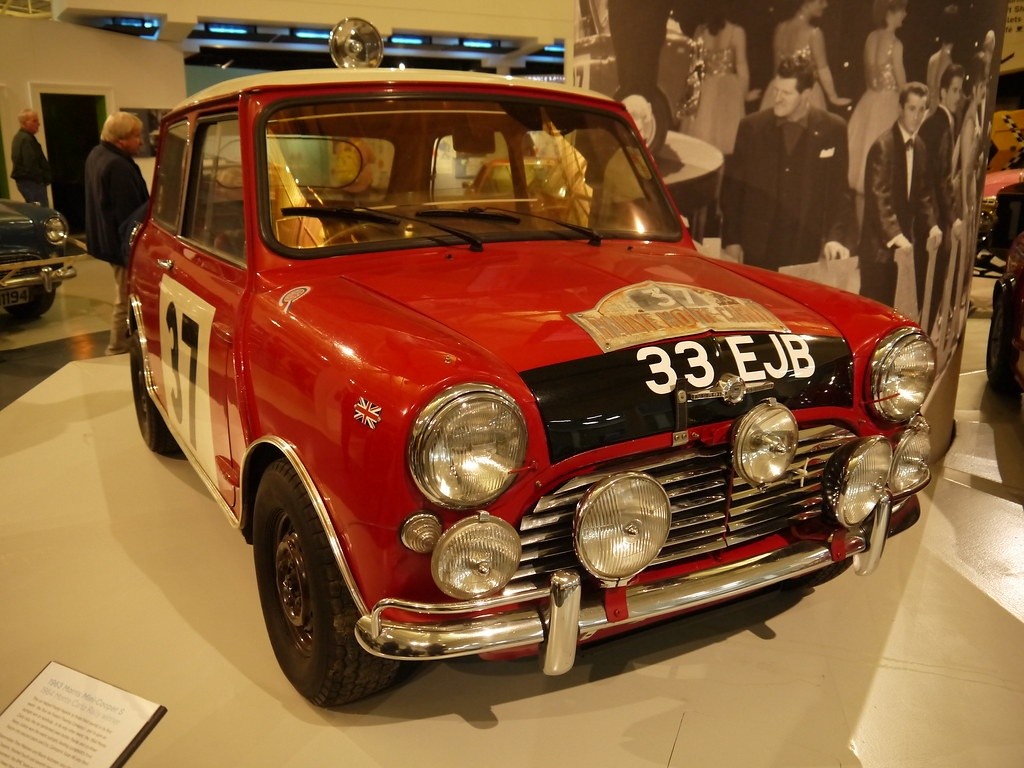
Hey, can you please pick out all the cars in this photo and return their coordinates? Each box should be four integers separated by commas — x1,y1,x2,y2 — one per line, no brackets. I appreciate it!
128,68,938,709
0,192,77,319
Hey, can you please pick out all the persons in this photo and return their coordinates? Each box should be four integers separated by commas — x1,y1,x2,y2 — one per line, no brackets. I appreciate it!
615,0,996,346
9,108,51,206
83,112,151,355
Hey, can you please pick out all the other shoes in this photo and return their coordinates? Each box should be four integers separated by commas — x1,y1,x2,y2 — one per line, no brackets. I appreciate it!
105,343,129,356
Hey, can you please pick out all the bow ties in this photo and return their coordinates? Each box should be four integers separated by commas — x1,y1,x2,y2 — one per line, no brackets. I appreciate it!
905,139,914,151
